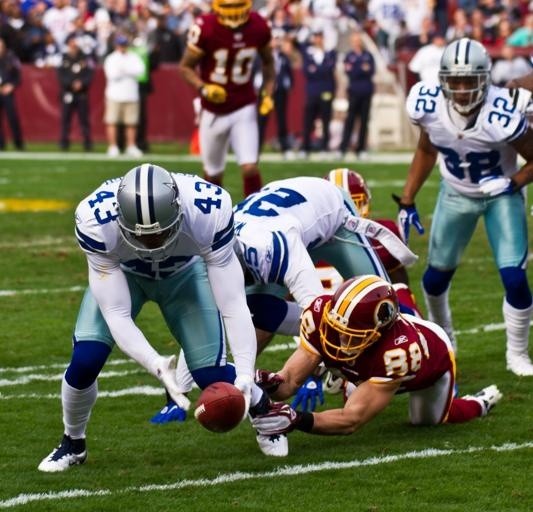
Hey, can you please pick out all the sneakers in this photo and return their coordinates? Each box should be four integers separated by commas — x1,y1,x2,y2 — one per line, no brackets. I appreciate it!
505,350,532,380
37,433,86,473
249,390,289,457
108,145,121,158
475,384,504,416
124,146,144,158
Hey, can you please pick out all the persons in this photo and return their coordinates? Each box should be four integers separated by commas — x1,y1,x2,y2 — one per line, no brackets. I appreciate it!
29,162,292,471
182,0,277,207
1,0,209,158
392,39,533,377
258,1,532,158
152,170,505,433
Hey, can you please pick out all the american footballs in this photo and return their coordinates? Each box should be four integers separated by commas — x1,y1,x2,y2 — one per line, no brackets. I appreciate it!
195,381,245,432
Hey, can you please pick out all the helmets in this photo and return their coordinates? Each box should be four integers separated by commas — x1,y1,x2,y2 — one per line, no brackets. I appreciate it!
210,0,252,26
116,164,182,263
319,275,400,363
323,168,372,218
439,38,493,112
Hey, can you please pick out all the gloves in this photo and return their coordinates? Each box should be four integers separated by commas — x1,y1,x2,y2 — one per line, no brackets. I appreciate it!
396,201,425,245
293,369,325,414
203,85,226,100
234,375,253,421
152,396,191,425
250,401,299,436
259,94,272,116
154,354,191,411
479,173,515,197
254,371,284,394
